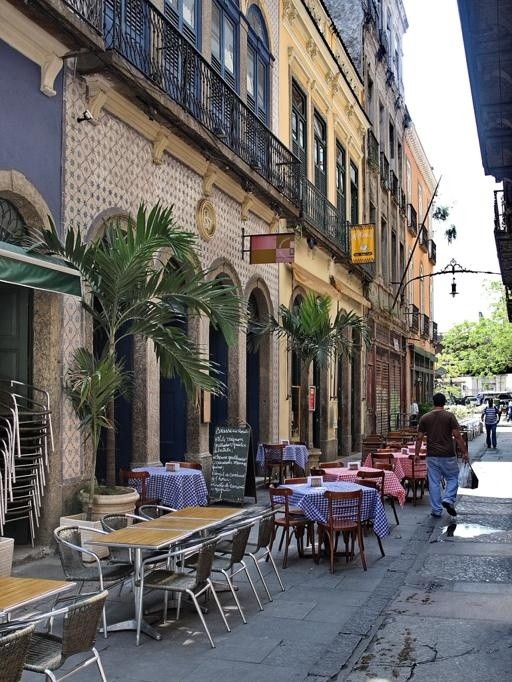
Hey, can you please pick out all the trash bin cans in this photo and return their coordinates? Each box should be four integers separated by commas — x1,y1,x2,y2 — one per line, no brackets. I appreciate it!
493,398,500,410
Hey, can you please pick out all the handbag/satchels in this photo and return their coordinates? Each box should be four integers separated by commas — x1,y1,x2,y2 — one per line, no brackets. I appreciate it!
471,470,478,489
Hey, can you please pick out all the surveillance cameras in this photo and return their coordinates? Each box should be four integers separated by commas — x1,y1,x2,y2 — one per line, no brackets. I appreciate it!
83,110,93,122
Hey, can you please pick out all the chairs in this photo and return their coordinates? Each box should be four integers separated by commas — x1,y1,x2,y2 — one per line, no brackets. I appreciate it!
40,524,133,639
282,422,472,575
22,588,110,681
175,522,265,626
99,503,181,598
215,506,285,603
0,622,36,680
1,373,57,549
133,533,232,650
119,458,209,513
257,440,311,484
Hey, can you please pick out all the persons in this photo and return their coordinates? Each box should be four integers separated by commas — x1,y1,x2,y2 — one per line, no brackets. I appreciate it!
481,398,501,449
505,400,512,422
411,400,420,426
413,392,470,518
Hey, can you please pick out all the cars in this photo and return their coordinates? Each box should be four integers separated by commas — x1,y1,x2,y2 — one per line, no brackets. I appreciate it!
452,390,512,406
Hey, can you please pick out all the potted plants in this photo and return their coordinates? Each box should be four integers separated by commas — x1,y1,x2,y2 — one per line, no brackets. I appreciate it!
250,294,378,474
4,196,250,534
55,345,136,566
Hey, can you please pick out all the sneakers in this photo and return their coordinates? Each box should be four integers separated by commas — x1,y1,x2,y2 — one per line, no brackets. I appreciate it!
487,445,497,449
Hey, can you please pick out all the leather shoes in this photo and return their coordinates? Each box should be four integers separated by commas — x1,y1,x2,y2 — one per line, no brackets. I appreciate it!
430,510,441,517
441,501,457,517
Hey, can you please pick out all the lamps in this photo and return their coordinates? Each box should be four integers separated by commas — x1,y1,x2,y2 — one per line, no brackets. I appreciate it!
449,275,460,299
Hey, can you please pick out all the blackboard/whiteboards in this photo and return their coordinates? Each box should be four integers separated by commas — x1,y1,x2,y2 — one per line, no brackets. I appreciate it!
207,426,250,502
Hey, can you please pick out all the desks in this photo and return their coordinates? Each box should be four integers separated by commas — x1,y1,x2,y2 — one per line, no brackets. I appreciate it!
1,574,78,620
82,505,253,641
